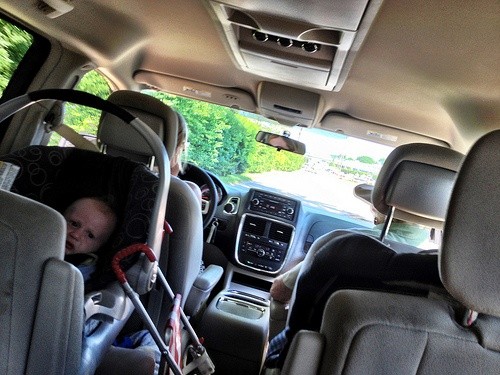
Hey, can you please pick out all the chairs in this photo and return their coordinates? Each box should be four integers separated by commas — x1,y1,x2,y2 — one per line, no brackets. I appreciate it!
96,91,224,358
266,129,500,375
0,189,84,375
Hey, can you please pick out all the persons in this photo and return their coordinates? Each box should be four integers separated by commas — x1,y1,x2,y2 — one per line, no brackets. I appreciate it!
270,182,430,302
151,111,202,201
267,134,298,154
65,195,117,281
